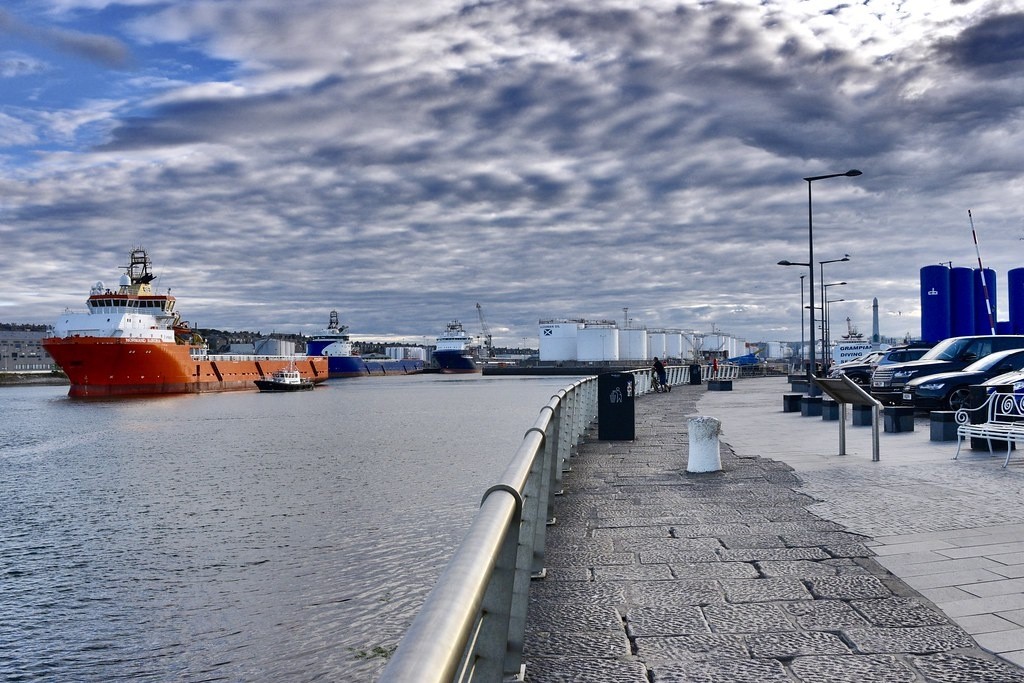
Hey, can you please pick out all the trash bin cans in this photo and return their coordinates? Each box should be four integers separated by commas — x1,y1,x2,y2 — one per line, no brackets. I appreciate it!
597,371,635,441
806,362,822,375
968,384,1016,450
690,364,702,385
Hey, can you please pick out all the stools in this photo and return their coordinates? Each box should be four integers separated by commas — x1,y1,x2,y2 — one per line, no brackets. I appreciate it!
884,407,914,433
852,404,873,427
801,396,823,417
930,411,965,441
822,399,846,421
791,380,808,392
783,394,803,412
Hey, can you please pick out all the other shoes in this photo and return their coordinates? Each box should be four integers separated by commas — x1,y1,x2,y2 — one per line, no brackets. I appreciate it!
663,390,667,392
668,386,671,392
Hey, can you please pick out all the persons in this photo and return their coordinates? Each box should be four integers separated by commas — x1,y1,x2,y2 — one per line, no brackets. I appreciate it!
651,357,672,392
713,359,718,379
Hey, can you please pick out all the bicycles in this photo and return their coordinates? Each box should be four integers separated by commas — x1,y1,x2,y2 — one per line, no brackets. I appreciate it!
651,376,664,393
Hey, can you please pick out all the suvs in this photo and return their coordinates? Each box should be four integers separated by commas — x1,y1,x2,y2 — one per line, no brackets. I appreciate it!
827,351,893,393
870,335,1024,412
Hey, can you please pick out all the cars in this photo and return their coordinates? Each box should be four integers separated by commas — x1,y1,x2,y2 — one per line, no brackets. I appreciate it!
980,367,1024,419
903,349,1024,418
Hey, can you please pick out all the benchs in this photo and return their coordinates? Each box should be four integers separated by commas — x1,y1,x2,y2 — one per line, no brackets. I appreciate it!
708,380,732,391
952,391,1024,468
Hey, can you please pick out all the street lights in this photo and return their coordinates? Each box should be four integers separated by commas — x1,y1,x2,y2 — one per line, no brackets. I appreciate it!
776,168,864,410
803,253,893,389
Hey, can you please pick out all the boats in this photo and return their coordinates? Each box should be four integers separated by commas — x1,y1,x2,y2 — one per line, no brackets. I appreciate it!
304,309,427,378
40,250,330,401
432,302,493,375
252,356,316,393
